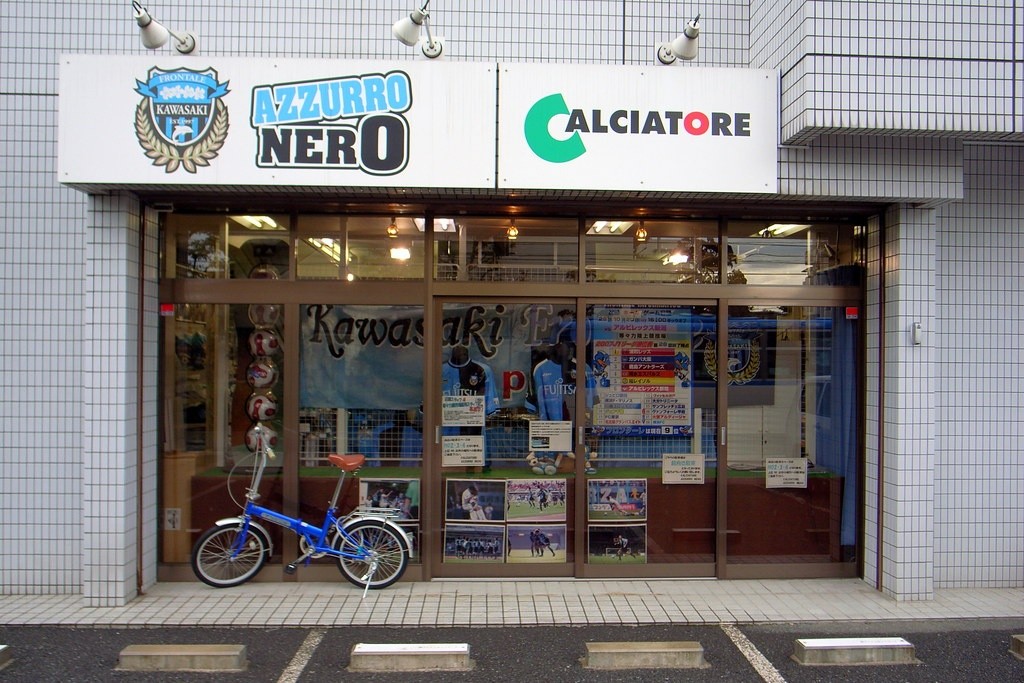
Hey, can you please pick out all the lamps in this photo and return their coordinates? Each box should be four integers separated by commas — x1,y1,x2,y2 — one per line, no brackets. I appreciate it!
635,219,648,242
391,0,445,61
506,218,518,239
657,13,701,65
387,217,399,238
824,244,836,259
130,0,195,54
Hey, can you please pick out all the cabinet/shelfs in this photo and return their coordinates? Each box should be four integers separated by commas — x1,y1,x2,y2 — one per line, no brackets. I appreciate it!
175,225,221,453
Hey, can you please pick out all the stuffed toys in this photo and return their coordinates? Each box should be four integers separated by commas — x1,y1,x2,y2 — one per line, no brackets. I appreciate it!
526,445,598,475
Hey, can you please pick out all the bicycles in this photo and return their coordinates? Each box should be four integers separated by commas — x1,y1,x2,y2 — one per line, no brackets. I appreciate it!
192,423,414,598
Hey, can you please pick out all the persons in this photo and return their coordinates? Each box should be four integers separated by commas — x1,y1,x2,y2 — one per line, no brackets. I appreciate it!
614,535,635,560
419,345,501,437
606,495,625,514
528,485,564,511
363,481,419,519
530,530,555,557
462,483,493,520
640,489,647,512
534,343,599,420
456,536,499,559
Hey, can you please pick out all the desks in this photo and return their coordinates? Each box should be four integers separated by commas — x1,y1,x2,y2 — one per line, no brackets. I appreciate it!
162,450,217,564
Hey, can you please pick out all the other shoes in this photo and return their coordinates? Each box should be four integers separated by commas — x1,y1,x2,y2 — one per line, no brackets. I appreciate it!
539,555,543,557
553,553,555,556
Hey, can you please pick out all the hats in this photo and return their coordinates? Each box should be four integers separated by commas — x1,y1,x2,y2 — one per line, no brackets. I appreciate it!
530,532,533,536
